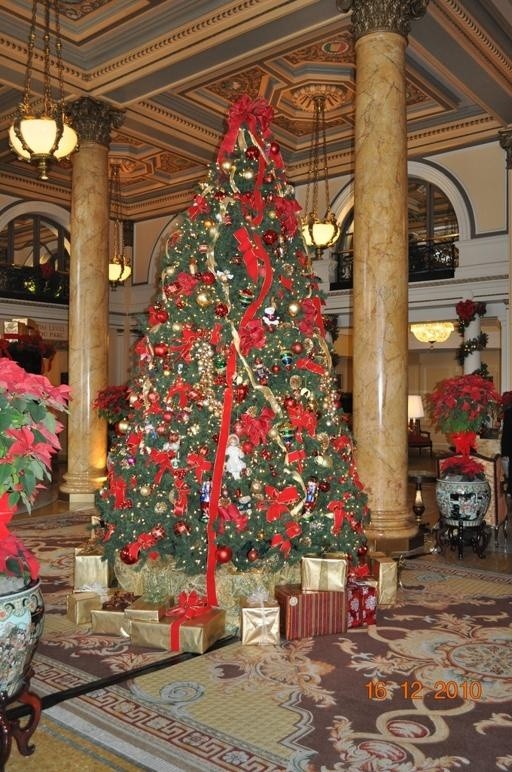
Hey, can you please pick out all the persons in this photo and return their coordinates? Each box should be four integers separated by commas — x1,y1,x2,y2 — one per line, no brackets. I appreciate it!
303,476,319,511
226,433,244,477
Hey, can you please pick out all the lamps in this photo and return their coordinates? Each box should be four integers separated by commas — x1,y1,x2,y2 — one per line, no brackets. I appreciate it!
109,162,133,287
8,2,82,181
302,94,341,262
411,321,455,346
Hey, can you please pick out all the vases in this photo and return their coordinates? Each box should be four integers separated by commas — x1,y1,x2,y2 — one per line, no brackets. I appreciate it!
435,476,492,527
0,572,43,699
115,423,126,437
479,428,499,440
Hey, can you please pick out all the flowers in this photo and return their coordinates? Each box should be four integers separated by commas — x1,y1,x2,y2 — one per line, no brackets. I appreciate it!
421,373,503,474
95,383,138,421
1,349,73,582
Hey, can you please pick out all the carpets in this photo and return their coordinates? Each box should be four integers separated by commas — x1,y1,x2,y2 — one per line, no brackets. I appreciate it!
2,508,511,770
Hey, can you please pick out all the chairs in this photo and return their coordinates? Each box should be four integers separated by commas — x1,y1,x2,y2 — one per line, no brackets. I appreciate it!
407,425,432,458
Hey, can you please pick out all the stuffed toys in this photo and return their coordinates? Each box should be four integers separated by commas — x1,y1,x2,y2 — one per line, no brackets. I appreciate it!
261,305,280,331
200,479,214,524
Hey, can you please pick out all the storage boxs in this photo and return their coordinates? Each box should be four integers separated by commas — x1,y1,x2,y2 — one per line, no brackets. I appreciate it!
274,584,347,641
240,594,281,647
130,609,225,654
5,316,40,344
368,551,397,605
345,583,377,629
90,598,131,637
300,552,349,592
346,561,371,578
124,593,174,623
73,551,116,591
68,591,118,625
